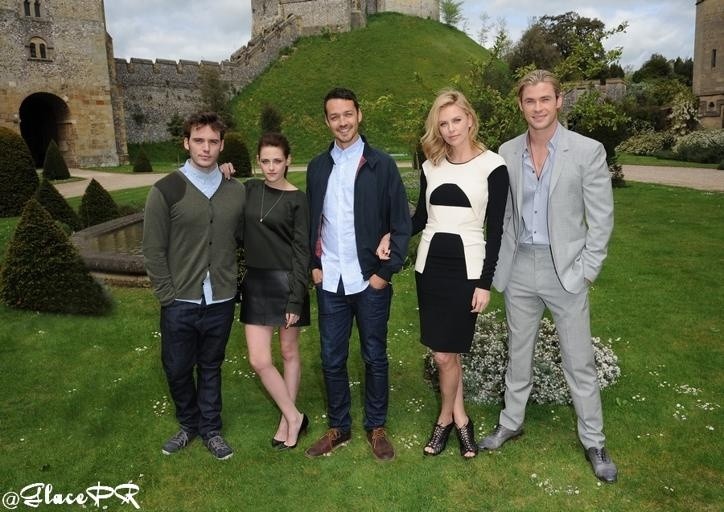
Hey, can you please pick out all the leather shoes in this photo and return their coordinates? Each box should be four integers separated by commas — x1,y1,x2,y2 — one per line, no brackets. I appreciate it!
477,426,523,453
584,446,618,484
272,438,285,448
364,428,394,460
279,413,309,451
305,428,352,459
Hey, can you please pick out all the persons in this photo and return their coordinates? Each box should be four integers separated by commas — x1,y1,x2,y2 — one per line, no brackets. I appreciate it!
377,87,510,460
304,87,412,463
477,67,620,485
139,109,247,463
218,131,315,452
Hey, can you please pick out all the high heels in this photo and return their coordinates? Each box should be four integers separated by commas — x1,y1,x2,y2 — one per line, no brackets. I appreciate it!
423,417,455,456
454,416,479,459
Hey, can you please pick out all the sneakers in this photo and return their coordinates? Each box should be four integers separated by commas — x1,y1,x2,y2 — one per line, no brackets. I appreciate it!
161,423,198,456
201,431,234,461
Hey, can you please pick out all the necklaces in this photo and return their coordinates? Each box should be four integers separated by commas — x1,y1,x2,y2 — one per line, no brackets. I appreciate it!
258,179,288,223
